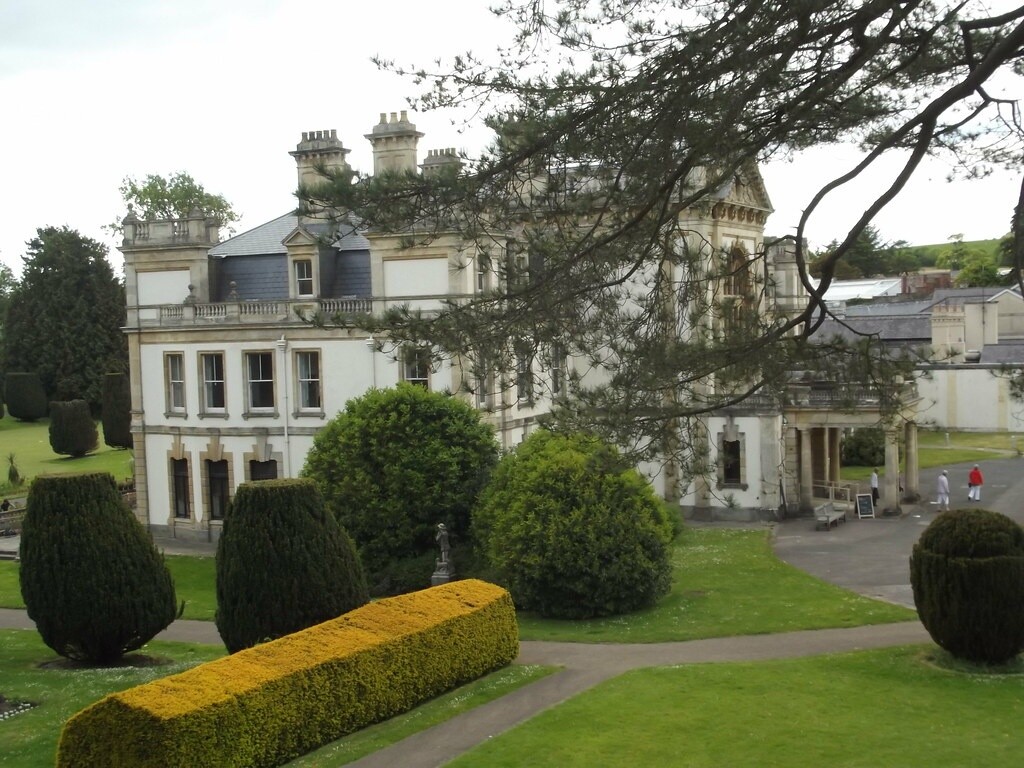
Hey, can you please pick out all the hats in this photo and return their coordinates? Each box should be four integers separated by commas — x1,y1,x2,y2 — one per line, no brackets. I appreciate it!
974,464,979,468
943,470,948,475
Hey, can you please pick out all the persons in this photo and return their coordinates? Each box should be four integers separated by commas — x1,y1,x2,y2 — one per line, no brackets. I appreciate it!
0,499,18,512
968,463,985,502
436,523,451,562
936,470,950,512
870,467,880,507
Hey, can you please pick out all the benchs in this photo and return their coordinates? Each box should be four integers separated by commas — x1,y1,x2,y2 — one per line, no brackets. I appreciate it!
814,502,847,531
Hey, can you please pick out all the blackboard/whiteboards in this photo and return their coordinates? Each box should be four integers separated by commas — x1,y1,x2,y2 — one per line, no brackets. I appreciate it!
856,494,874,516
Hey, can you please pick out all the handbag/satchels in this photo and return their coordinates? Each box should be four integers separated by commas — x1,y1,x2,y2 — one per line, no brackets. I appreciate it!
968,483,971,487
